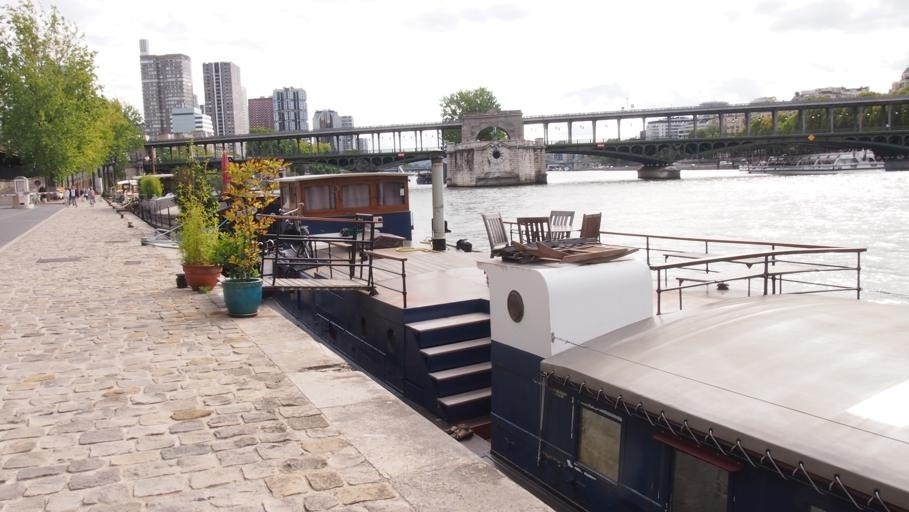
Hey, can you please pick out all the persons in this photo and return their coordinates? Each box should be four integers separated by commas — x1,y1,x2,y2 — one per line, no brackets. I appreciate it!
63,184,96,209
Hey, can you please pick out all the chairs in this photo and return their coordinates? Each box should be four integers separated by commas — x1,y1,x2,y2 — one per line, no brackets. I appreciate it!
480,209,602,259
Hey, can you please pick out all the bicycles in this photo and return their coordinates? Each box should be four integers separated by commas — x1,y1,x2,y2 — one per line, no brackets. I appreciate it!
278,201,314,259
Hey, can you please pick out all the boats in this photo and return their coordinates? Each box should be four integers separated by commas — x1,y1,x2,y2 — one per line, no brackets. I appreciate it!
764,149,886,171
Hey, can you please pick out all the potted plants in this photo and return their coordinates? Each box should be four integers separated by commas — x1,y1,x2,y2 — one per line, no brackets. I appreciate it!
171,138,295,318
